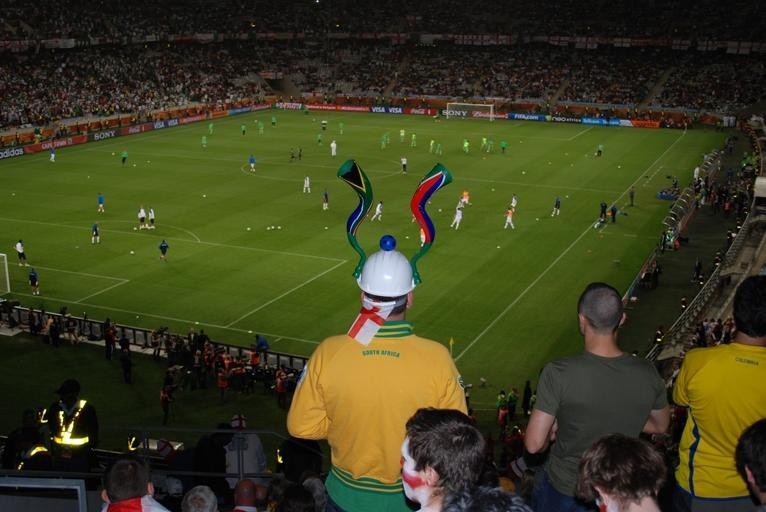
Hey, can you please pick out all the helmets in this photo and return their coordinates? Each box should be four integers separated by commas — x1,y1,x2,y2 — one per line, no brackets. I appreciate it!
356,250,417,298
